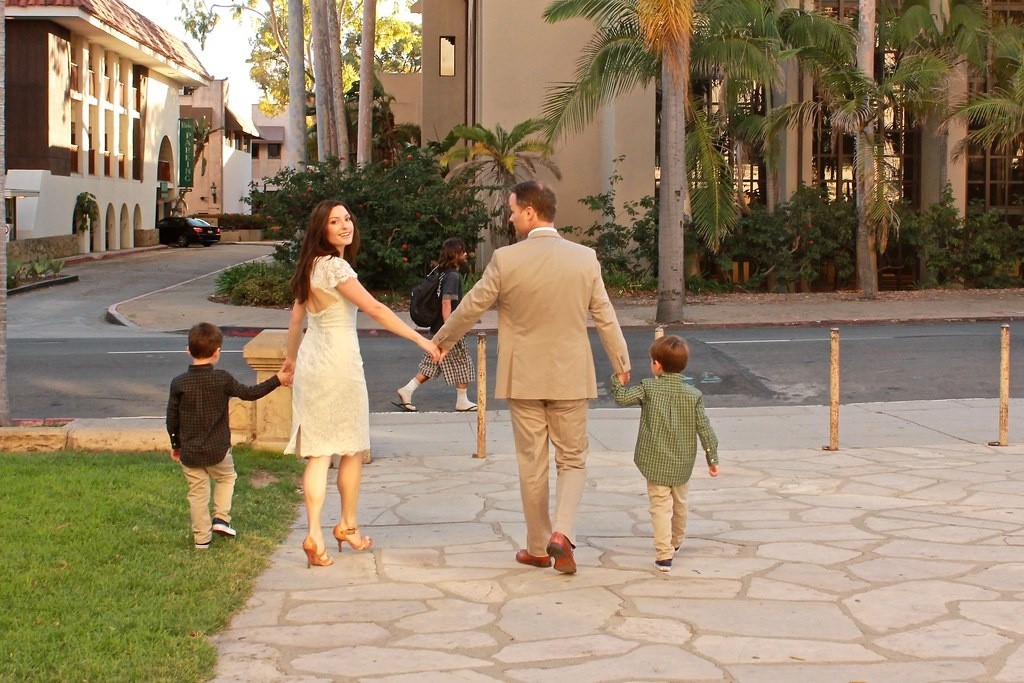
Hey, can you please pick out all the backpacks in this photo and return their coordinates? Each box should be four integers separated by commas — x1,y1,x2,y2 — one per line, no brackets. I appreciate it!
410,267,460,327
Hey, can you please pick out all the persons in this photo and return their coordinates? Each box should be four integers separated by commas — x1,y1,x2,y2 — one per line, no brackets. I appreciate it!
611,334,720,571
279,199,440,569
391,237,478,411
166,322,292,549
431,181,631,573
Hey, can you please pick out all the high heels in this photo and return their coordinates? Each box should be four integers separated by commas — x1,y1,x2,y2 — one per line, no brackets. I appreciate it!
302,535,334,569
333,525,374,552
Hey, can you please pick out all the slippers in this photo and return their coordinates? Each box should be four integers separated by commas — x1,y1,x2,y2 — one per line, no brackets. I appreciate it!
391,400,418,413
457,404,477,411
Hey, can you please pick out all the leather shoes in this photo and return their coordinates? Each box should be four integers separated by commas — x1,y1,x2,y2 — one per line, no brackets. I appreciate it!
517,550,551,567
547,532,577,574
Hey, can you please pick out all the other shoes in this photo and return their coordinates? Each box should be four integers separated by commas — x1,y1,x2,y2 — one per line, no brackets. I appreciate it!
674,546,680,552
655,561,672,572
212,518,237,536
195,543,210,549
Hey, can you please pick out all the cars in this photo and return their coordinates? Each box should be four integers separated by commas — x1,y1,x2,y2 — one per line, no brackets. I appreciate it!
155,215,222,248
